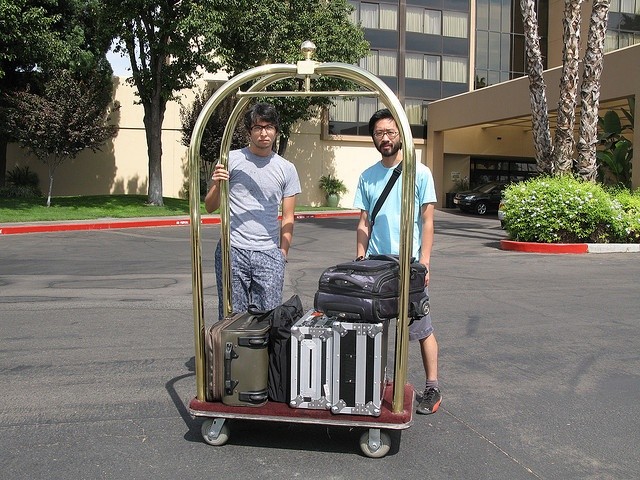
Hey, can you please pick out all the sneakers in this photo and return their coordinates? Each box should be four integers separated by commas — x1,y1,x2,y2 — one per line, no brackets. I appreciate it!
416,388,443,415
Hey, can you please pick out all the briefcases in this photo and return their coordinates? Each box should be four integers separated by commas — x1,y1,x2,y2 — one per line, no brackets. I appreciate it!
290,309,333,410
331,319,391,417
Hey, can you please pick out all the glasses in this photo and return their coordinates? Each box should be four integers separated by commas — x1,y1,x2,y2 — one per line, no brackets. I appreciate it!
373,128,402,138
250,124,277,132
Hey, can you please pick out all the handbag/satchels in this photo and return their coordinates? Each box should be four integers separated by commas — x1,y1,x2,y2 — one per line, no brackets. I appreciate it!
248,294,305,403
368,160,402,237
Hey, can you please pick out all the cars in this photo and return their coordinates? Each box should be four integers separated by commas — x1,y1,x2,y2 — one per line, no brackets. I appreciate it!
454,181,507,214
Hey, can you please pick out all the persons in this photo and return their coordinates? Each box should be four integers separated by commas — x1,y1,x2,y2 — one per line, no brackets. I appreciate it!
355,108,443,416
205,105,302,322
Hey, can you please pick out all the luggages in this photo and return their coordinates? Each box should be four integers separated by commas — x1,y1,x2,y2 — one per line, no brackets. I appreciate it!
314,254,430,324
205,312,270,407
